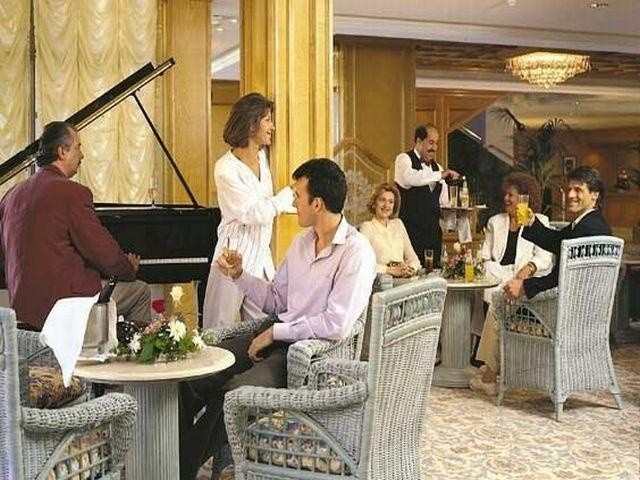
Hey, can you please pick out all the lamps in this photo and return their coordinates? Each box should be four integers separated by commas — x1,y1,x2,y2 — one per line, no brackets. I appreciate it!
495,47,598,89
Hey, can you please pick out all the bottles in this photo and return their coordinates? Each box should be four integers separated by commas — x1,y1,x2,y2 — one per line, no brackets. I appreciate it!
440,244,449,272
97,274,120,303
465,249,475,283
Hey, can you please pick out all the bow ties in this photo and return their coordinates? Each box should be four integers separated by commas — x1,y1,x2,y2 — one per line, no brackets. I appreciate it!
419,157,430,166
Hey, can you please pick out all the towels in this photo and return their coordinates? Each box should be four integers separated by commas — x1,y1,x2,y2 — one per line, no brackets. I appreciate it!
444,211,472,244
39,291,118,389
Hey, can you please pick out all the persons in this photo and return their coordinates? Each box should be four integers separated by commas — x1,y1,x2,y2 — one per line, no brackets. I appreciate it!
360,182,422,282
203,92,298,335
394,124,459,268
468,166,612,395
0,121,151,332
480,171,553,305
179,157,378,480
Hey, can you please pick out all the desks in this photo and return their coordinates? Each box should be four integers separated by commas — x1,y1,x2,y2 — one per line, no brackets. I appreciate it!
611,259,640,344
73,344,235,479
431,275,500,387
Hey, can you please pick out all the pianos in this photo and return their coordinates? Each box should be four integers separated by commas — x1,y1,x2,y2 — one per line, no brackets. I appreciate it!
1,58,221,284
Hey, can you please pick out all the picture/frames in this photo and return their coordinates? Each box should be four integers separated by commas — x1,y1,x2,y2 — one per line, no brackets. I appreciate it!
564,155,576,176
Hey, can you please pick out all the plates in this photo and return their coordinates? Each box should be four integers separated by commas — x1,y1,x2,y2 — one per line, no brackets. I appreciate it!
440,206,487,211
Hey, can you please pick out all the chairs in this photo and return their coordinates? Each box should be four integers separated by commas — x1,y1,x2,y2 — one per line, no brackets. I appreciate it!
0,308,139,479
496,236,626,423
202,299,368,480
223,276,448,479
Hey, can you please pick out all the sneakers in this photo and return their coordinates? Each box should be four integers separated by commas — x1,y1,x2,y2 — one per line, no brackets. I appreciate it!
469,375,497,397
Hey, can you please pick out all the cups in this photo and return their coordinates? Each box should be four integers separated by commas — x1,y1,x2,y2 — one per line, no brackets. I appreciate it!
224,237,237,288
516,195,529,226
424,250,433,274
450,186,458,209
